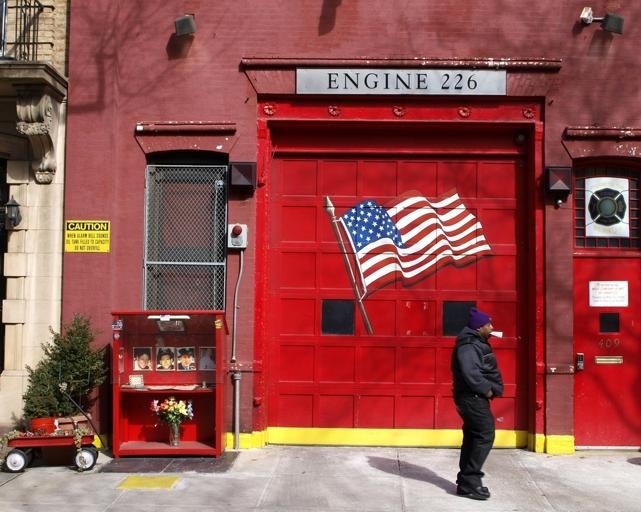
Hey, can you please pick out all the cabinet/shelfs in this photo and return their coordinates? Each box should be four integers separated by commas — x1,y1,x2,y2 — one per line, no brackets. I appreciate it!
109,308,230,458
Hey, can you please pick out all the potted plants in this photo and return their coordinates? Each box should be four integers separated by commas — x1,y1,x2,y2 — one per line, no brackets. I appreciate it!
42,312,108,430
22,363,60,434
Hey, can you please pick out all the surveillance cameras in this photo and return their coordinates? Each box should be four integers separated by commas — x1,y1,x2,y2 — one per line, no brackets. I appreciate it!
580,7,593,24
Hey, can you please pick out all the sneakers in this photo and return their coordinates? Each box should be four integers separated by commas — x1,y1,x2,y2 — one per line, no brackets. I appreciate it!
457,484,491,499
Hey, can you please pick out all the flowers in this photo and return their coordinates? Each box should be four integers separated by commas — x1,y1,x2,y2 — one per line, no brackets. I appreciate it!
150,396,194,437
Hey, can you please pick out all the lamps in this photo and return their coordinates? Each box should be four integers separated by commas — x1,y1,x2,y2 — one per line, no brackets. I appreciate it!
5,195,21,228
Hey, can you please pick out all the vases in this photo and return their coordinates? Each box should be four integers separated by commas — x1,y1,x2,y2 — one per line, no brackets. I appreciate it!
168,422,180,448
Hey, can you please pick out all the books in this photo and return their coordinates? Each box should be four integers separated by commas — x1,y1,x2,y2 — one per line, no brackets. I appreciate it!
148,384,200,390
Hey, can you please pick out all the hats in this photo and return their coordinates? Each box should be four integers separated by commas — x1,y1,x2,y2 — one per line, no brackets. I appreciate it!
467,307,493,329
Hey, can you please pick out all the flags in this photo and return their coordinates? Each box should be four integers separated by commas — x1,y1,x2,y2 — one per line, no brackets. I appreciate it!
340,186,496,295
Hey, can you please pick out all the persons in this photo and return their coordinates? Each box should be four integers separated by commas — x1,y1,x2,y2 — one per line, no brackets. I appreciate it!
176,347,196,370
156,348,174,369
199,349,215,368
134,350,152,370
450,307,503,500
